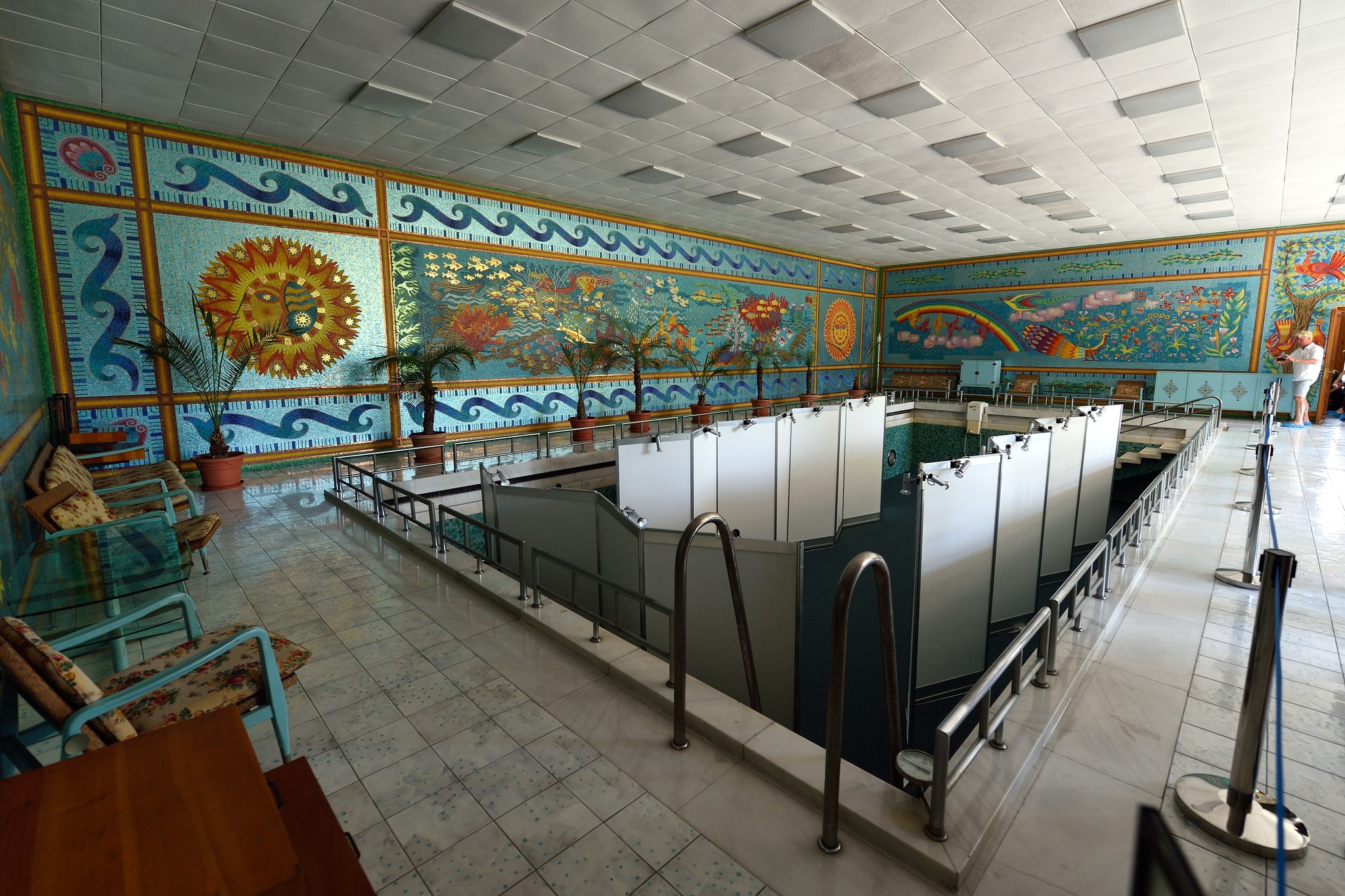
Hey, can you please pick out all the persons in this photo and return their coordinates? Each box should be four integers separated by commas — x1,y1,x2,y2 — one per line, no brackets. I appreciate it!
1274,331,1324,428
1325,349,1345,421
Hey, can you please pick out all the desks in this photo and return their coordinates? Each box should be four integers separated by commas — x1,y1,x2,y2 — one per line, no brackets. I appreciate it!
957,384,1001,405
6,520,191,674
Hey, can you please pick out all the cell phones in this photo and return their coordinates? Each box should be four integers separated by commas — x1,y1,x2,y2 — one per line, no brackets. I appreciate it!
1276,358,1286,361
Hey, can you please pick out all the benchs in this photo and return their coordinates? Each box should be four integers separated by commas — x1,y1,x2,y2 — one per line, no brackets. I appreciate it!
22,440,195,513
881,371,959,402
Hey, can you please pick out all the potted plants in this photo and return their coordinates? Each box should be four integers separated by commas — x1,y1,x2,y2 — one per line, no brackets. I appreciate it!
130,278,301,490
822,332,891,401
598,310,680,433
793,346,818,409
557,324,621,441
662,336,736,425
738,339,783,417
367,301,477,463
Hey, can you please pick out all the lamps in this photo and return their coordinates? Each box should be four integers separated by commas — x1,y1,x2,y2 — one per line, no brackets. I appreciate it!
901,403,1106,495
492,469,510,487
716,528,740,538
652,397,873,452
616,504,648,528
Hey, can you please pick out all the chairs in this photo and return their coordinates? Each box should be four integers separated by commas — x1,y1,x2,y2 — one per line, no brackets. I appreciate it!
0,593,314,764
22,478,222,575
1005,374,1040,406
1108,380,1147,414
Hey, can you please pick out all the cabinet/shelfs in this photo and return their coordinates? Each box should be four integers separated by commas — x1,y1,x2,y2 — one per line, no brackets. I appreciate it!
0,705,377,896
1153,370,1296,420
